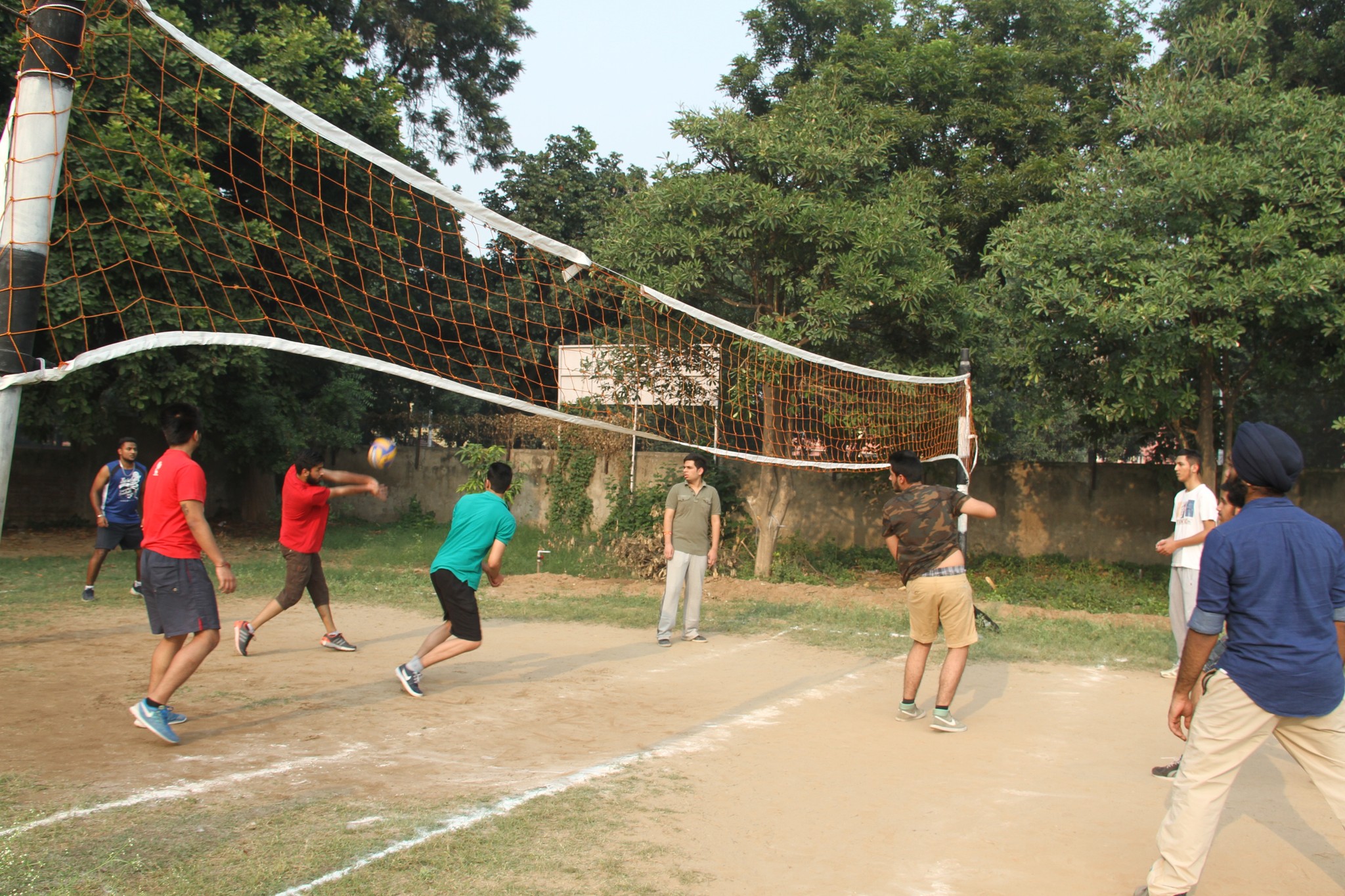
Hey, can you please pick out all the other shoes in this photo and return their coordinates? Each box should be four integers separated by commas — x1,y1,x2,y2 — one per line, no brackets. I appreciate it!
658,639,671,647
1160,659,1180,678
692,636,707,643
1134,886,1188,896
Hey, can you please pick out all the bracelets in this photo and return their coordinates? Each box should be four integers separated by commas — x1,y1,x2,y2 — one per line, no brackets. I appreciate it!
96,515,103,517
214,562,231,568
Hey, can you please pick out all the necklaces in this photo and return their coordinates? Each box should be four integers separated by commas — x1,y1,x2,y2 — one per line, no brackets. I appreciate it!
119,460,135,476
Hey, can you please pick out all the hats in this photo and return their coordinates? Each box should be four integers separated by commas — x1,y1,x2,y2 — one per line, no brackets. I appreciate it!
1233,420,1304,491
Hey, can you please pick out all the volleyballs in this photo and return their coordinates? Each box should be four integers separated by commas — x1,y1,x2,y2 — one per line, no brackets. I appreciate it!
367,438,397,474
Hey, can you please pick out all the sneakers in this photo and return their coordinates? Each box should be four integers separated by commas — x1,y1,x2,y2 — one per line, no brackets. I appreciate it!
1151,755,1182,778
82,588,95,602
129,583,144,600
929,709,967,732
320,633,357,651
394,663,424,697
234,620,254,656
133,705,187,728
895,702,926,722
129,698,181,743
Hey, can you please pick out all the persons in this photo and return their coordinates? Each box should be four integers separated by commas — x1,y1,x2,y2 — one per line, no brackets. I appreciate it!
128,403,237,745
234,448,388,657
395,462,517,698
1134,421,1345,896
80,437,148,601
1151,448,1241,780
882,451,996,731
657,453,722,647
